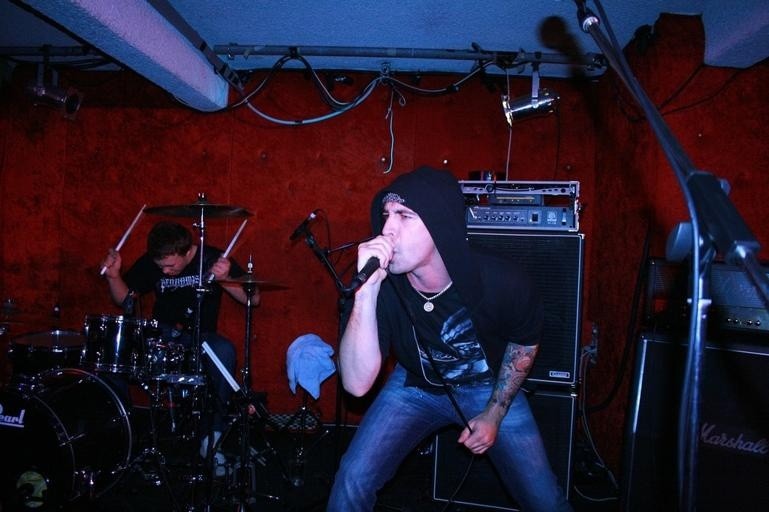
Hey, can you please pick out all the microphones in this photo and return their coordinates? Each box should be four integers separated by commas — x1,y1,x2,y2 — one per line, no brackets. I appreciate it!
289,208,320,241
350,243,391,296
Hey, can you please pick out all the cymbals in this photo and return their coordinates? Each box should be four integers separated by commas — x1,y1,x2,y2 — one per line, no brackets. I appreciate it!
144,203,254,218
209,280,289,292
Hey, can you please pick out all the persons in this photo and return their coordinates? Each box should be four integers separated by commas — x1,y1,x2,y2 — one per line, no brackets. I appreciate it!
101,221,261,477
325,165,578,511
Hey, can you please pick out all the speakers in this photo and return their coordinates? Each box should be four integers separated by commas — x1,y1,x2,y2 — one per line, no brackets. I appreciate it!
465,230,587,393
624,328,769,510
429,390,587,512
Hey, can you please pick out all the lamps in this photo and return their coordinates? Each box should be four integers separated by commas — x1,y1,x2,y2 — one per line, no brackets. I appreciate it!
22,45,83,123
499,52,562,128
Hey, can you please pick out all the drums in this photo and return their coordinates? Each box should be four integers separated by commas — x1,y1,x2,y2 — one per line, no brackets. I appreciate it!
11,330,83,370
146,335,208,386
83,313,162,374
13,366,132,503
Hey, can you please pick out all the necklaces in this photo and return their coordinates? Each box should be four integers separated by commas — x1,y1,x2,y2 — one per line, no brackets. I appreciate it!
411,281,453,312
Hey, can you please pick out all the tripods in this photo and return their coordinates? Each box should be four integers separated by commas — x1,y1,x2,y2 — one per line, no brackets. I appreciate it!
118,205,289,511
